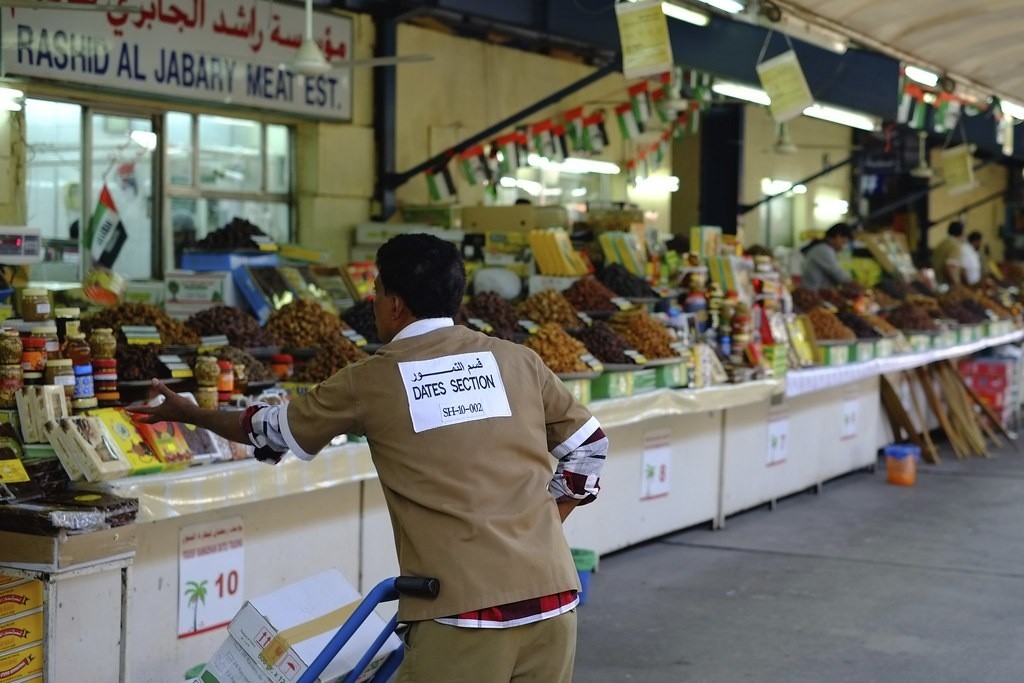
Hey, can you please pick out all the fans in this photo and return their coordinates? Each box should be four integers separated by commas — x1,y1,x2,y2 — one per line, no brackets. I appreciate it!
201,0,436,79
730,121,860,155
903,132,971,183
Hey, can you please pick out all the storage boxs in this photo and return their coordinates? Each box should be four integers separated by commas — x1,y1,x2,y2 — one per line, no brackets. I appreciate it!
162,271,248,306
148,391,222,466
352,247,380,262
126,279,164,307
165,304,211,321
59,415,132,482
401,205,461,230
181,251,279,269
30,385,67,442
278,242,327,265
307,265,359,302
131,403,195,471
461,205,1024,431
0,523,139,683
228,566,403,683
348,261,379,300
198,634,272,683
90,407,162,477
237,265,308,321
15,386,40,443
43,418,85,481
353,221,464,245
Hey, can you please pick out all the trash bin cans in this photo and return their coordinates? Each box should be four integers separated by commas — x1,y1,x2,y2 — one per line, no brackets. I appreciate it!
885,445,919,486
570,548,596,605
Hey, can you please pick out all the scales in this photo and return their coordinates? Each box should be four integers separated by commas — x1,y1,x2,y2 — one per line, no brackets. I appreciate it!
0,226,81,291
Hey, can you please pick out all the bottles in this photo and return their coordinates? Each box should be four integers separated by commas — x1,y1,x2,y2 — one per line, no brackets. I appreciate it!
0,330,23,365
196,387,219,410
89,328,116,358
21,288,52,322
62,331,91,366
35,326,60,362
194,355,221,386
46,359,74,396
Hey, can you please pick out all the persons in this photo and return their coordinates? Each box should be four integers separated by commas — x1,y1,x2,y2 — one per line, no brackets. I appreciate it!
800,223,866,293
929,220,965,286
958,229,983,293
125,232,609,683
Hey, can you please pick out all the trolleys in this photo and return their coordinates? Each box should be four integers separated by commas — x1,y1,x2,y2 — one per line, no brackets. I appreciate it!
293,575,440,683
